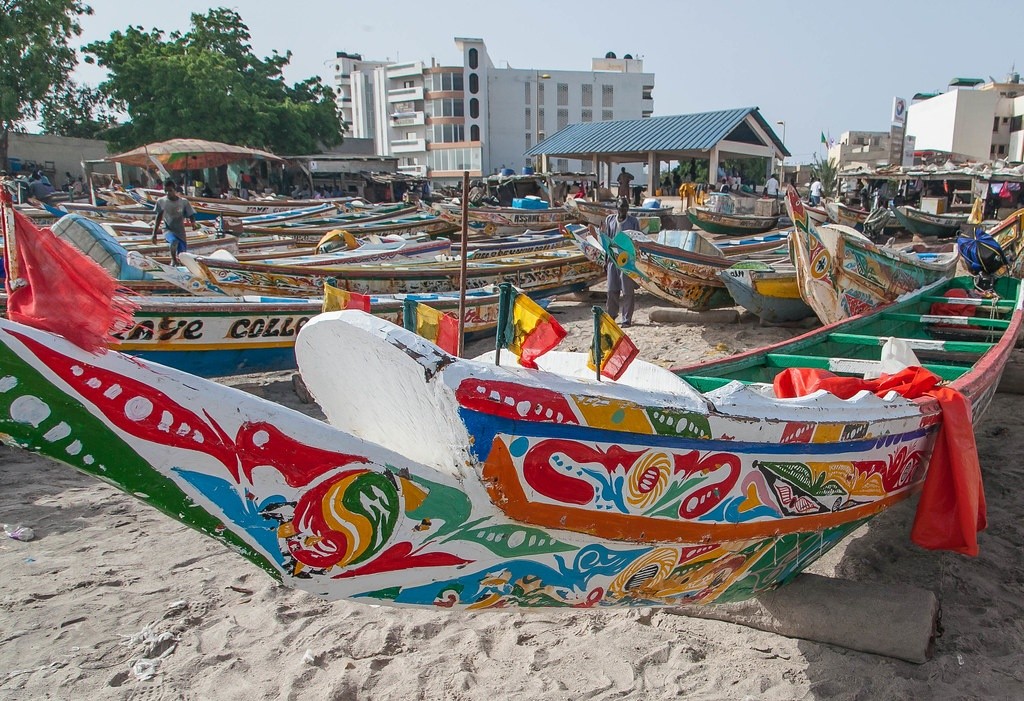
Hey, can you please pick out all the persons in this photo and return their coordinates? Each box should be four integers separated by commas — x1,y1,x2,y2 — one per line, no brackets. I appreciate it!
600,197,640,329
236,170,248,198
764,174,779,198
673,172,681,187
154,178,212,198
27,170,54,207
808,178,826,207
314,185,343,199
617,166,634,203
152,181,197,266
663,177,672,189
61,171,75,192
717,161,726,181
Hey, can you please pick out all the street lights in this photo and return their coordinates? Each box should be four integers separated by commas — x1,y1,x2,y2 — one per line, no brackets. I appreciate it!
778,120,786,189
536,70,553,170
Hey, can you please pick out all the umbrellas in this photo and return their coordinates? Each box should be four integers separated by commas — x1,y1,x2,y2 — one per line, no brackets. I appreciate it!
105,138,283,195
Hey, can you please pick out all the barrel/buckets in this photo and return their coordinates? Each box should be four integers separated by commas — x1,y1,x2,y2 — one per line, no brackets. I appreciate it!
501,168,514,176
522,166,534,175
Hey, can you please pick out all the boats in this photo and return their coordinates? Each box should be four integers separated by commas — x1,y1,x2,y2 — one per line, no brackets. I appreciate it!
0,190,1022,607
2,170,1023,382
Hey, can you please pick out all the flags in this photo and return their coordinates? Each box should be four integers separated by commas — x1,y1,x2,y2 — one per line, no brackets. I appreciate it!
821,133,828,148
403,299,459,356
496,283,566,371
586,306,638,380
322,285,371,314
